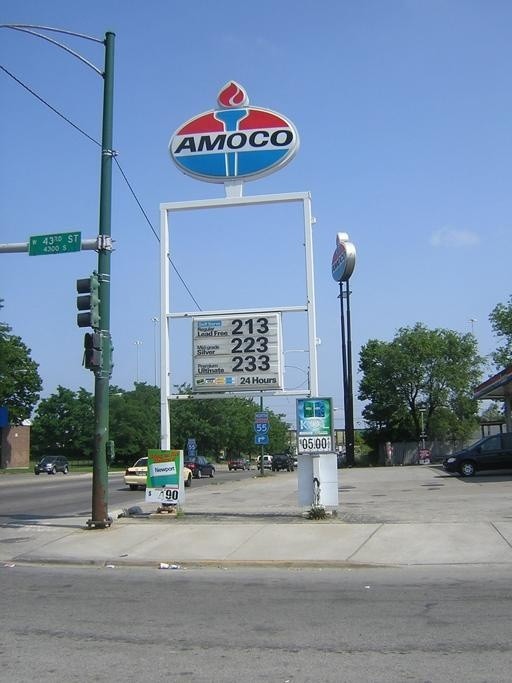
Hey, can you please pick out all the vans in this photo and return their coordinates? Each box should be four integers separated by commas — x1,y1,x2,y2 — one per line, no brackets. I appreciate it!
258,453,295,472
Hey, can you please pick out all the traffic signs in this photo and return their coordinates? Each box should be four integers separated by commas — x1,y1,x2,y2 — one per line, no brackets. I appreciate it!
254,419,270,435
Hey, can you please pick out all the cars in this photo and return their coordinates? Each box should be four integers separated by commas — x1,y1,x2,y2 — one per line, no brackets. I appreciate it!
124,456,193,491
228,456,251,471
442,432,512,477
184,455,216,479
34,456,69,475
